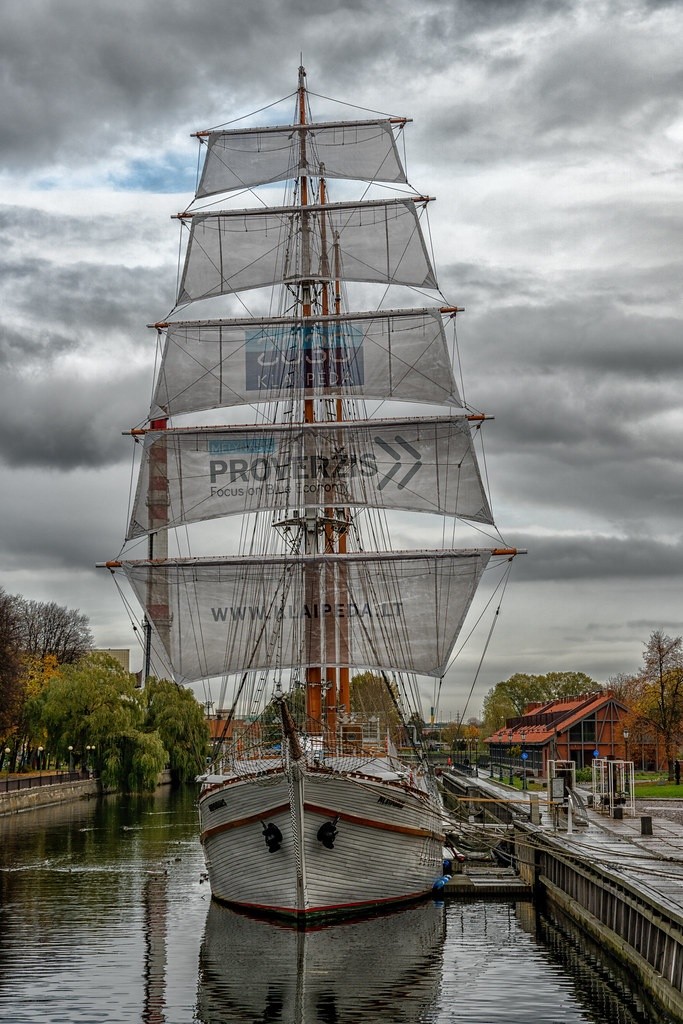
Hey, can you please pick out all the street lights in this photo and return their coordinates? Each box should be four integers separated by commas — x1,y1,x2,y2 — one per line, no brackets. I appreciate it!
5,744,10,792
498,732,503,781
86,745,95,779
520,731,528,789
488,734,493,779
507,730,514,784
452,736,472,775
38,744,44,786
67,744,73,782
623,729,630,792
474,737,480,778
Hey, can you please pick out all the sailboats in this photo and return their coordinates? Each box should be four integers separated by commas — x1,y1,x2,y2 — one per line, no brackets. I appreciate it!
196,900,446,1024
92,50,530,926
